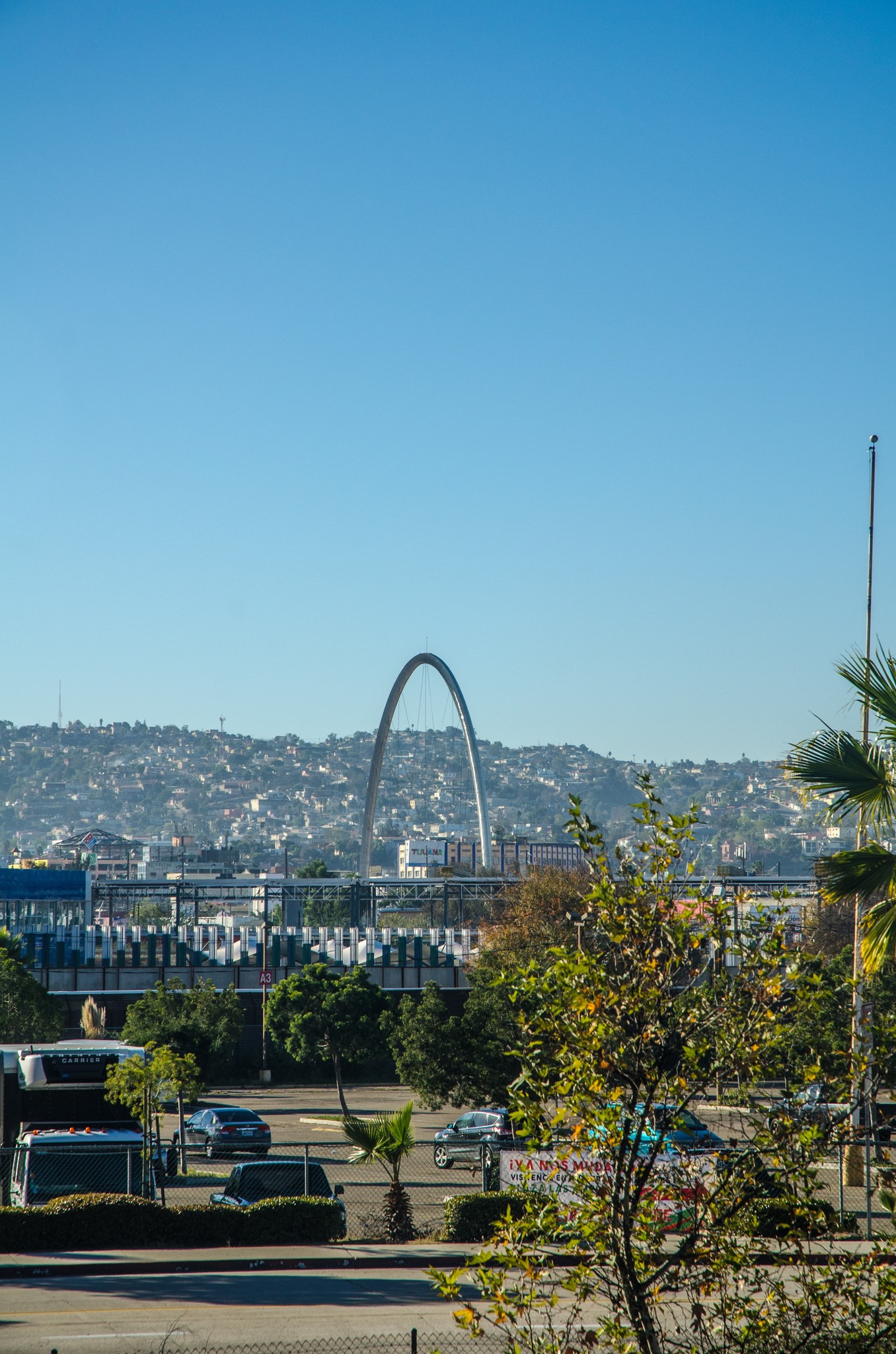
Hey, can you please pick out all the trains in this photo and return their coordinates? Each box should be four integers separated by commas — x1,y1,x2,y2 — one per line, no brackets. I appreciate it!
43,985,477,1075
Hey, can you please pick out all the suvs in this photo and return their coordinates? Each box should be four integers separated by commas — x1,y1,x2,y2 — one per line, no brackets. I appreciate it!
433,1104,555,1172
584,1100,726,1158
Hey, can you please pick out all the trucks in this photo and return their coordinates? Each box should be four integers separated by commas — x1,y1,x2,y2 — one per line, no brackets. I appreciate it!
0,1037,178,1207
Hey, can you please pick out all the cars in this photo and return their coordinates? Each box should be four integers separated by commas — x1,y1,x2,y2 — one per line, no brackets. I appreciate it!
210,1160,350,1238
152,1075,178,1105
172,1107,273,1162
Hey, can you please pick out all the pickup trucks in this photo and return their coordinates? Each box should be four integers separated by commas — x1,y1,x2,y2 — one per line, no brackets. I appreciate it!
764,1083,896,1143
